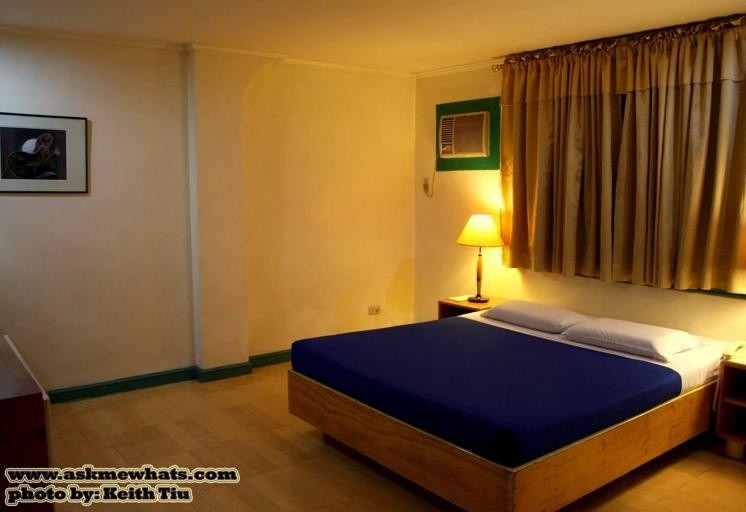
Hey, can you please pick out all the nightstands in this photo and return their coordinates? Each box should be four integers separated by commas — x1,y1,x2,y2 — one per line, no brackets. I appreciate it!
438,297,492,320
715,364,746,445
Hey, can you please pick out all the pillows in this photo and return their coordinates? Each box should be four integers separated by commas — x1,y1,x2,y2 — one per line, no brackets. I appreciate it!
481,298,702,362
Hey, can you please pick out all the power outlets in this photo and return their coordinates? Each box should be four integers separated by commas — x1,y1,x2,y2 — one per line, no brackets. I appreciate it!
422,176,429,193
368,305,380,316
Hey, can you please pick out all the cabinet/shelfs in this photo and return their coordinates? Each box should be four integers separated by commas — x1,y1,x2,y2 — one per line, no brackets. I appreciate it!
0,334,54,512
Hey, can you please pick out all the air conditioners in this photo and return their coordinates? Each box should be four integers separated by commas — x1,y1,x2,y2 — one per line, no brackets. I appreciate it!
437,109,491,158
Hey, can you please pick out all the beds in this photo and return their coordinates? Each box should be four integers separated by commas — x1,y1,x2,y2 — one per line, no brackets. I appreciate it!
289,308,727,512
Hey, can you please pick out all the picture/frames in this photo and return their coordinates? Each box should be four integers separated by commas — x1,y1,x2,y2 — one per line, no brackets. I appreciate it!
0,111,88,193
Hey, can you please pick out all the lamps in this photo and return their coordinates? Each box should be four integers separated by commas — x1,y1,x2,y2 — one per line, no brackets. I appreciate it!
456,213,503,303
